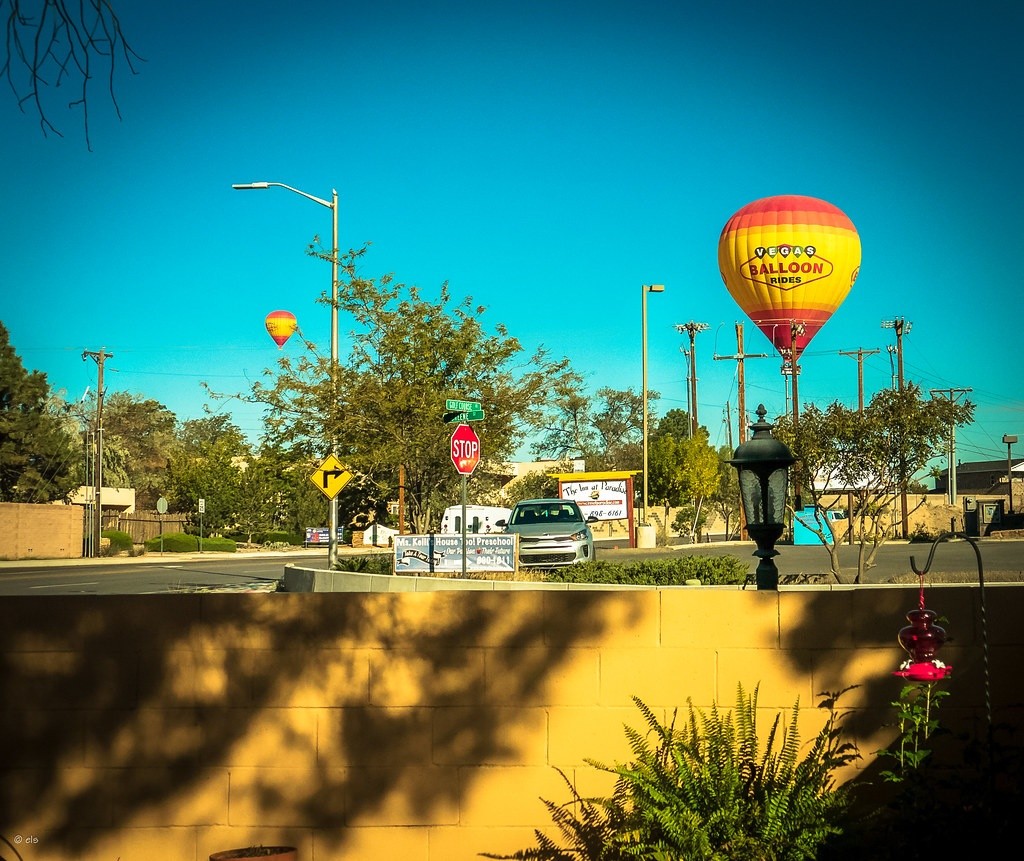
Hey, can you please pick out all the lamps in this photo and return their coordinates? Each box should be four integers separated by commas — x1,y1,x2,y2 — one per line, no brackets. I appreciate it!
892,567,953,682
724,405,800,559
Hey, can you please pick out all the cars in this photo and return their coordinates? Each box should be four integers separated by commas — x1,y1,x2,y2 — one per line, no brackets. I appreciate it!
496,498,599,573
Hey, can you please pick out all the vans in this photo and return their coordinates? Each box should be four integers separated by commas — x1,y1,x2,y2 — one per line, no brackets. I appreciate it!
441,504,512,534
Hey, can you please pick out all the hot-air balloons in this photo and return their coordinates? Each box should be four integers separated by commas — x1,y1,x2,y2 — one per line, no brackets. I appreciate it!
265,311,297,351
718,196,863,375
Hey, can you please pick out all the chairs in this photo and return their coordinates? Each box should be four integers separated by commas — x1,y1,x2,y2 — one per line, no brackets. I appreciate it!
557,510,569,522
524,511,538,524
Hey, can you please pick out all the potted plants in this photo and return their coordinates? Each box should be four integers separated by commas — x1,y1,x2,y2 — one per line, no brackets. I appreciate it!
101,529,134,557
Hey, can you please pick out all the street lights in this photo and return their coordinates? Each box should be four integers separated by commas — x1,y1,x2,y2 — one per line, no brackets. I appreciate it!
232,183,339,572
1002,432,1019,513
634,284,665,526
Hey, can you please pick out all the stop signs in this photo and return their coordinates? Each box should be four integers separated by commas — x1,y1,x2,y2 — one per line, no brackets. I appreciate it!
450,424,481,475
